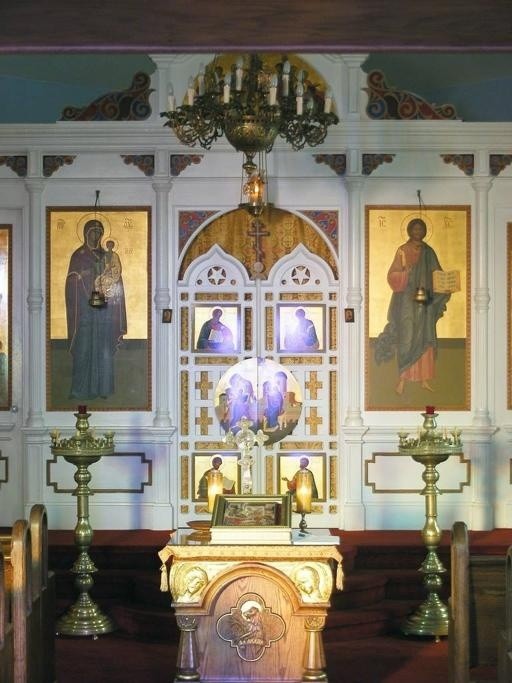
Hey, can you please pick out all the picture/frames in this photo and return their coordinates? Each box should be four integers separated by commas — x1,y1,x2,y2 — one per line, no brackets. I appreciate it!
191,452,241,504
364,203,473,413
191,303,242,355
44,204,153,414
211,493,293,529
276,452,328,505
276,303,327,354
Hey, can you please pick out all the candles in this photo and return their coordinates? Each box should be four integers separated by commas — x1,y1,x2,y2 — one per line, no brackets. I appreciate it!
296,472,313,513
208,472,223,513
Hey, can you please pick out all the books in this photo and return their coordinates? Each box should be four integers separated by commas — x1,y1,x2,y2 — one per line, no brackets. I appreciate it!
433,266,462,294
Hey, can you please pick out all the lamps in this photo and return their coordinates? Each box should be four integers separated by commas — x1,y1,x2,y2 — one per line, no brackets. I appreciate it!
158,51,339,219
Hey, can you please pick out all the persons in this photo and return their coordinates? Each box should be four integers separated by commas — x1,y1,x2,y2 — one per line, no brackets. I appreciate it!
192,307,235,353
283,306,318,351
195,457,236,495
94,239,123,301
63,216,130,404
373,217,452,396
281,456,318,500
